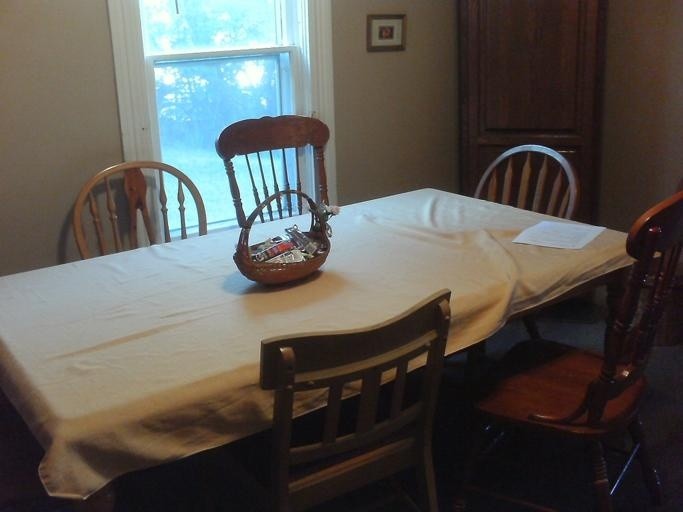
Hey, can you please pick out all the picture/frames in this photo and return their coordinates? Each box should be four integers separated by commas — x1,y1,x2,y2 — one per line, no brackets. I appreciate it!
365,14,407,53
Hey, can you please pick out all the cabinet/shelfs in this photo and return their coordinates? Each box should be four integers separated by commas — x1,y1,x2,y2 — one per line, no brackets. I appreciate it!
453,0,605,218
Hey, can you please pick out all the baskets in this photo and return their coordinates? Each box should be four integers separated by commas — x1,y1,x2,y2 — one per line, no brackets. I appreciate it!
233,190,331,286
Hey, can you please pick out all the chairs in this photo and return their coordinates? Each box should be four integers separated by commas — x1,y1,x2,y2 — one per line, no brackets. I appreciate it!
452,189,683,512
70,160,206,260
214,115,330,226
470,143,583,357
246,288,451,510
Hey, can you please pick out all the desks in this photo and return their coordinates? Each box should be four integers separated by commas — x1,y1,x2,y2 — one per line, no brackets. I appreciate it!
0,187,643,512
